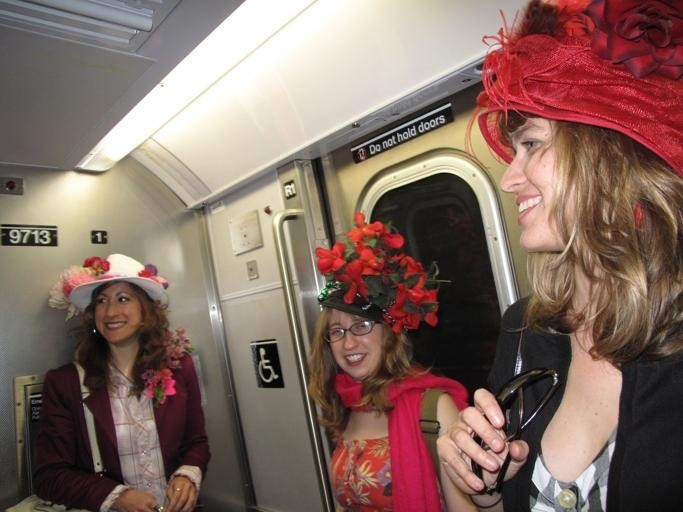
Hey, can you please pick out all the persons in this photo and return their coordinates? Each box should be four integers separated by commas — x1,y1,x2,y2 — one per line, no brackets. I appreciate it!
309,305,469,512
437,108,682,512
30,251,211,512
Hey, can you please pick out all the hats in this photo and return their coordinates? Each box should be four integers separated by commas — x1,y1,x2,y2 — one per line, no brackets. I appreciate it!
321,290,394,326
477,1,682,178
71,253,169,311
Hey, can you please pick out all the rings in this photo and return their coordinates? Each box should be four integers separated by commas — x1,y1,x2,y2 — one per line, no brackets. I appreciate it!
174,487,183,491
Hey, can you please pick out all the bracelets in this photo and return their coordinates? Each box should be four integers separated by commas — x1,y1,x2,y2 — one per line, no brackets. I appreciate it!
468,495,503,509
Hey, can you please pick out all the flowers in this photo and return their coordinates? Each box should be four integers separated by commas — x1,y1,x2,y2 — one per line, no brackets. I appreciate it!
313,211,452,334
576,0,683,81
139,325,195,405
47,253,169,322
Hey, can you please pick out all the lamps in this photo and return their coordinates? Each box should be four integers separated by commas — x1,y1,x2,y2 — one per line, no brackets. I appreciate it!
69,0,319,172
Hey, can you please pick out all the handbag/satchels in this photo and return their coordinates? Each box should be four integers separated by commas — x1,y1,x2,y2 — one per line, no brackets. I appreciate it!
1,494,91,512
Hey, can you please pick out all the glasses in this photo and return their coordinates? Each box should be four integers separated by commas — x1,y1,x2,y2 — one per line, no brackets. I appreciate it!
470,367,565,494
321,319,379,342
